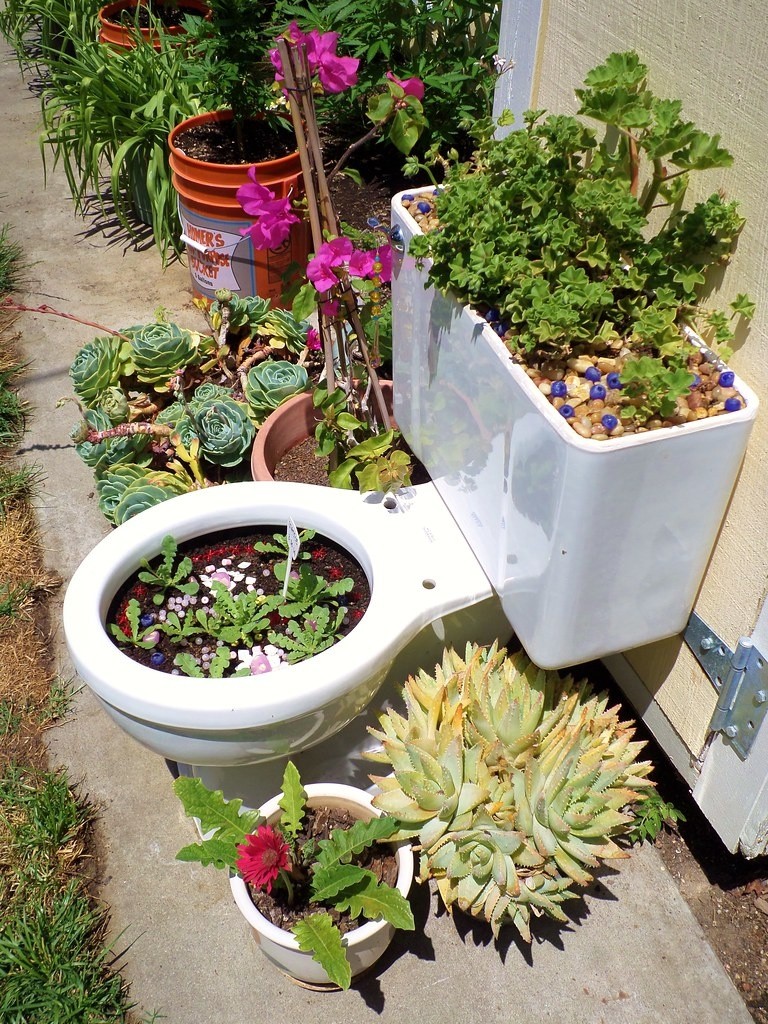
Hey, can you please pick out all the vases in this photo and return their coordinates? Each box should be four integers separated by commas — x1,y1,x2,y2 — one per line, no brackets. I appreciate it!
99,0,213,57
249,379,432,492
226,783,413,991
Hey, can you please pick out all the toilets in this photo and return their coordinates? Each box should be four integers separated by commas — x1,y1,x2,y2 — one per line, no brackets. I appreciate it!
61,163,757,844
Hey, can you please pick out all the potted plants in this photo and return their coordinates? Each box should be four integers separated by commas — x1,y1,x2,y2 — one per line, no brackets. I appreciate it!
359,637,659,944
167,0,310,314
393,49,757,672
61,480,513,841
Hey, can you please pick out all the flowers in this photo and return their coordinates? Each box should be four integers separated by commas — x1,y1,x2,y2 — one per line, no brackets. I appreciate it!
172,758,416,991
237,20,424,494
